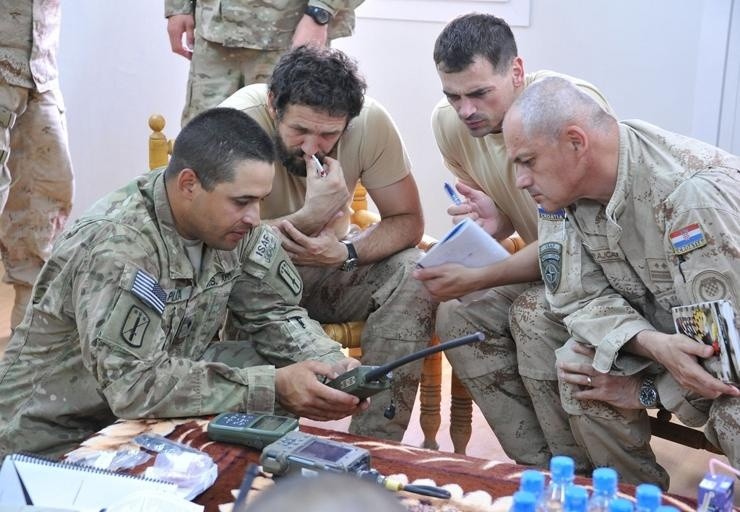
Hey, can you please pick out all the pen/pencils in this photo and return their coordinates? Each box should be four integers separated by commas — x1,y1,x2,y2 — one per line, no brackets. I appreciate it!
312,155,355,216
445,183,461,206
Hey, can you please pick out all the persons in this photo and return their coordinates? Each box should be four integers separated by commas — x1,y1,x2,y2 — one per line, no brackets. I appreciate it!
501,76,740,512
0,2,74,342
1,109,373,475
412,13,615,484
165,0,367,137
214,42,438,443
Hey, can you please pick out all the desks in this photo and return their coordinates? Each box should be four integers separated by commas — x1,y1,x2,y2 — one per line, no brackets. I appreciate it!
58,401,740,512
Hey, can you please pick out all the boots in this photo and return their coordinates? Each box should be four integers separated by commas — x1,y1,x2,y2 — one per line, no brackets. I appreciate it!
10,284,32,330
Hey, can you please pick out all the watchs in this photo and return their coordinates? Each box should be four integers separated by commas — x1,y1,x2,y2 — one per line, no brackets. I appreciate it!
638,374,660,409
304,6,330,25
339,239,360,273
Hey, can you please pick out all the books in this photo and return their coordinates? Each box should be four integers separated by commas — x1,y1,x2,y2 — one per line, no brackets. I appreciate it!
1,454,175,511
415,217,515,305
666,297,740,390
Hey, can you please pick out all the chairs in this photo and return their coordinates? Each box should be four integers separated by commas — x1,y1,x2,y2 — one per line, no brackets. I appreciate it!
148,114,443,456
447,227,725,465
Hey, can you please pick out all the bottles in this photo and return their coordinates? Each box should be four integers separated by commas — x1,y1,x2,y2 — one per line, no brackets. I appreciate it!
510,458,682,512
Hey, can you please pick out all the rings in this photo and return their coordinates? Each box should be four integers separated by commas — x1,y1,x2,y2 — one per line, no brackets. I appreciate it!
587,375,592,386
